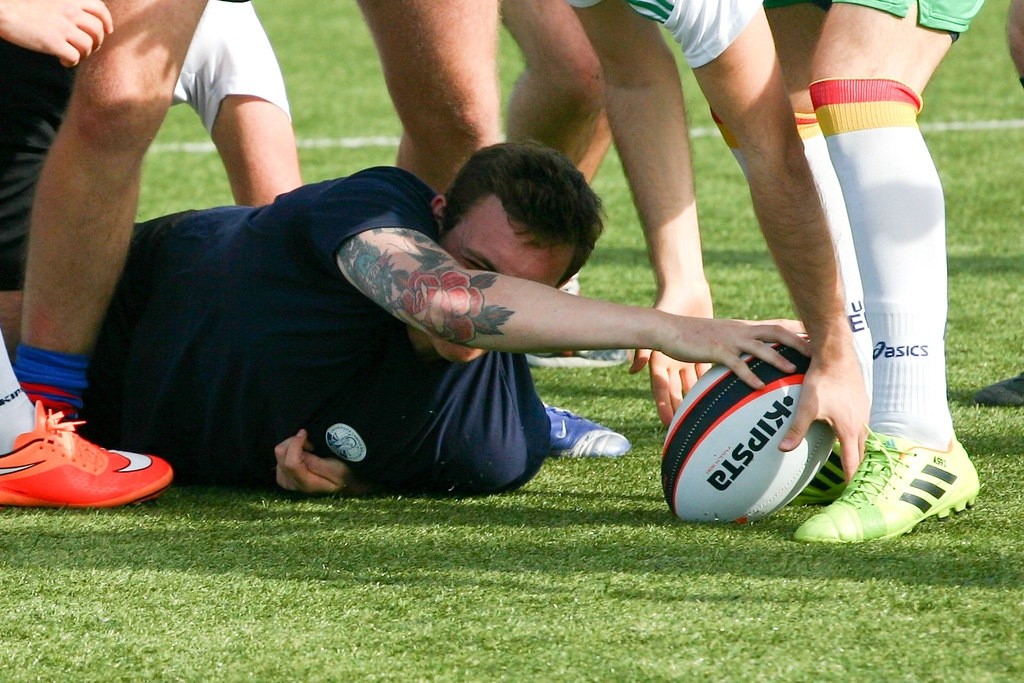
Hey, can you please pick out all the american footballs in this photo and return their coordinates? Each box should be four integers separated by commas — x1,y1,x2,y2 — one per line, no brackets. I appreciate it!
659,333,840,525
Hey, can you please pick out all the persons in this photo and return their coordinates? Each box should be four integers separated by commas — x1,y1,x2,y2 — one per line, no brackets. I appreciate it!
80,143,814,497
0,0,1022,546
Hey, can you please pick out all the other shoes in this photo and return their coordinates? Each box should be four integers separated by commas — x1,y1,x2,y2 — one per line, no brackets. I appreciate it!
974,372,1024,407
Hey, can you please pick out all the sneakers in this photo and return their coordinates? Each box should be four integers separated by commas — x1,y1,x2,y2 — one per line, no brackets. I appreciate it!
524,350,629,368
792,422,980,545
0,400,174,508
787,441,845,504
543,401,632,458
